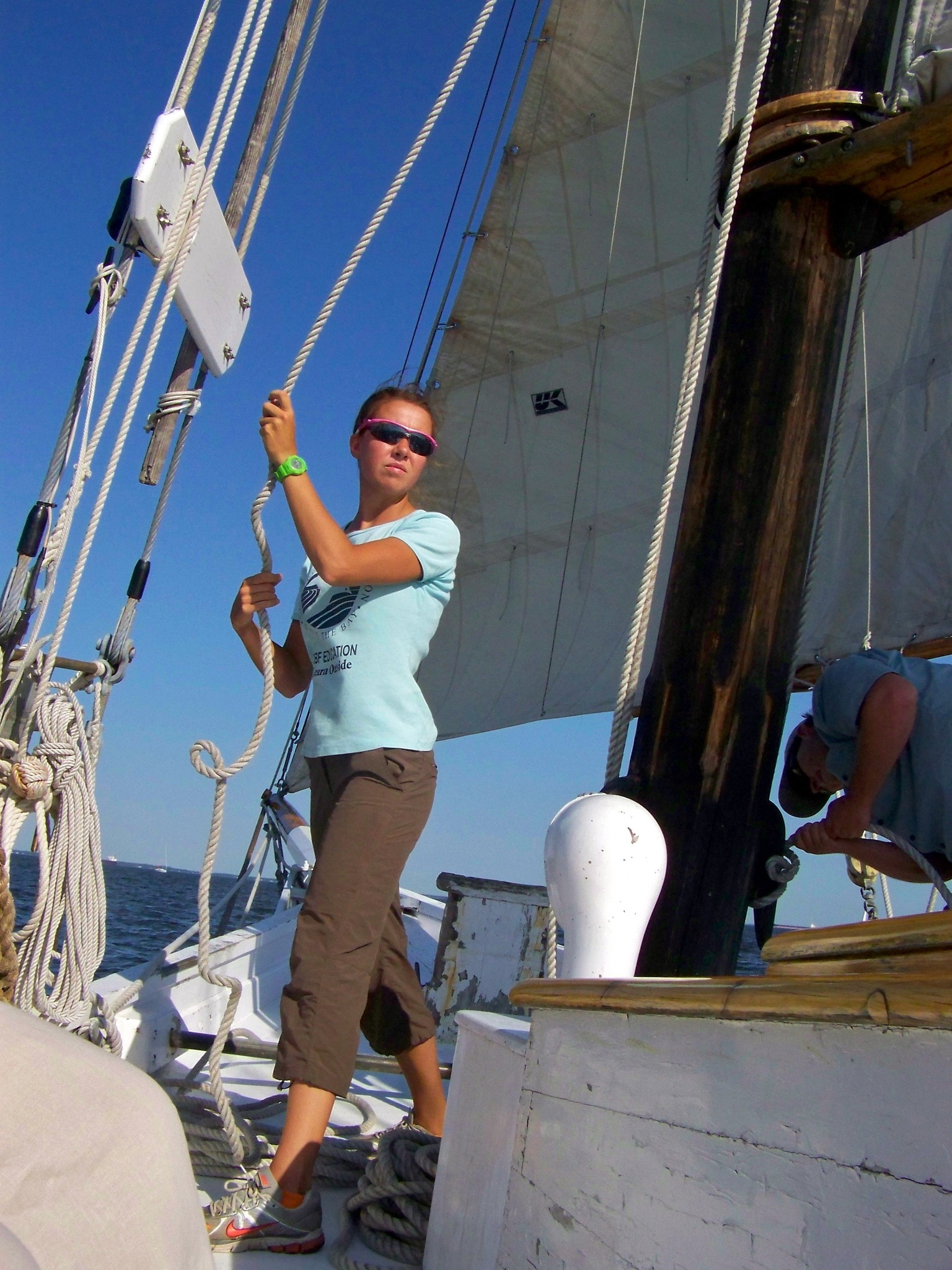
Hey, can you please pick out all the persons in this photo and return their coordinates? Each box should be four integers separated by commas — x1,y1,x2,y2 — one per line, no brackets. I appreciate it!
203,385,462,1255
777,647,952,883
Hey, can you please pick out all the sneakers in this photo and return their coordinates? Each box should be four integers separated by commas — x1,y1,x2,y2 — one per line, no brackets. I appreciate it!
203,1164,324,1254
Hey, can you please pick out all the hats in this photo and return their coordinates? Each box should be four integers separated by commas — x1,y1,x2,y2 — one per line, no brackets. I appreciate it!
778,718,832,817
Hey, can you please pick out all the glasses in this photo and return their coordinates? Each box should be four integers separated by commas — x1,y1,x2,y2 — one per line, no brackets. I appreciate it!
354,419,437,458
793,758,832,801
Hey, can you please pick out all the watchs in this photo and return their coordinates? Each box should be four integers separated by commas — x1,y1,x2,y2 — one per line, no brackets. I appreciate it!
274,455,308,484
